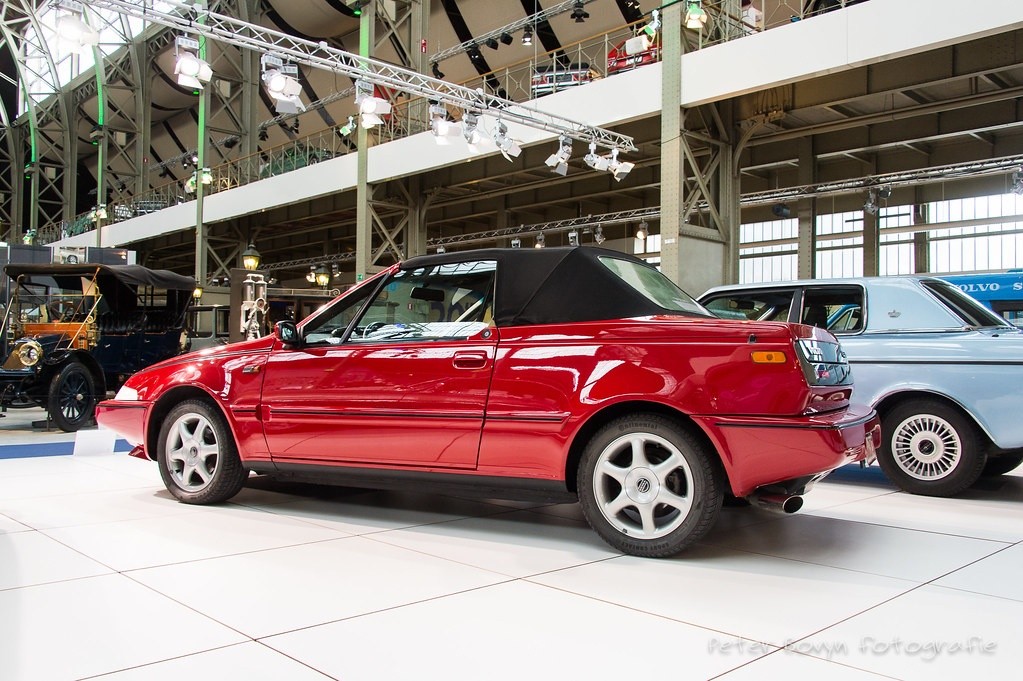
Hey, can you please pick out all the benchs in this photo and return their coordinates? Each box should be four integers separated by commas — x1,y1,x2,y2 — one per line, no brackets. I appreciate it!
98,304,182,336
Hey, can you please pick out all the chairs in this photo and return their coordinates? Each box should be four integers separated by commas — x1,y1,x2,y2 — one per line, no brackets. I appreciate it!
804,305,827,329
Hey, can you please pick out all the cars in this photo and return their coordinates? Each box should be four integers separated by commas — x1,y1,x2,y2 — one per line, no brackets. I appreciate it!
0,260,198,434
691,272,1023,498
94,244,885,560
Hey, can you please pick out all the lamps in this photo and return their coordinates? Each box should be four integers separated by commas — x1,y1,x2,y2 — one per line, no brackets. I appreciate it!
353,1,362,15
486,38,498,50
261,52,306,113
258,126,268,141
624,0,640,9
288,116,300,134
242,239,262,270
571,0,589,22
182,154,198,170
685,0,707,29
432,62,445,80
52,0,100,54
511,219,606,248
354,79,391,129
638,10,661,46
173,32,213,89
863,185,891,215
429,102,449,137
118,183,126,192
87,204,107,222
224,138,238,148
490,118,524,163
500,33,513,45
522,24,532,45
462,110,478,144
193,277,205,332
340,115,357,136
468,40,483,62
159,166,169,178
545,134,573,176
1011,170,1023,195
583,139,608,170
306,261,343,286
184,168,212,193
603,149,635,183
637,218,649,239
